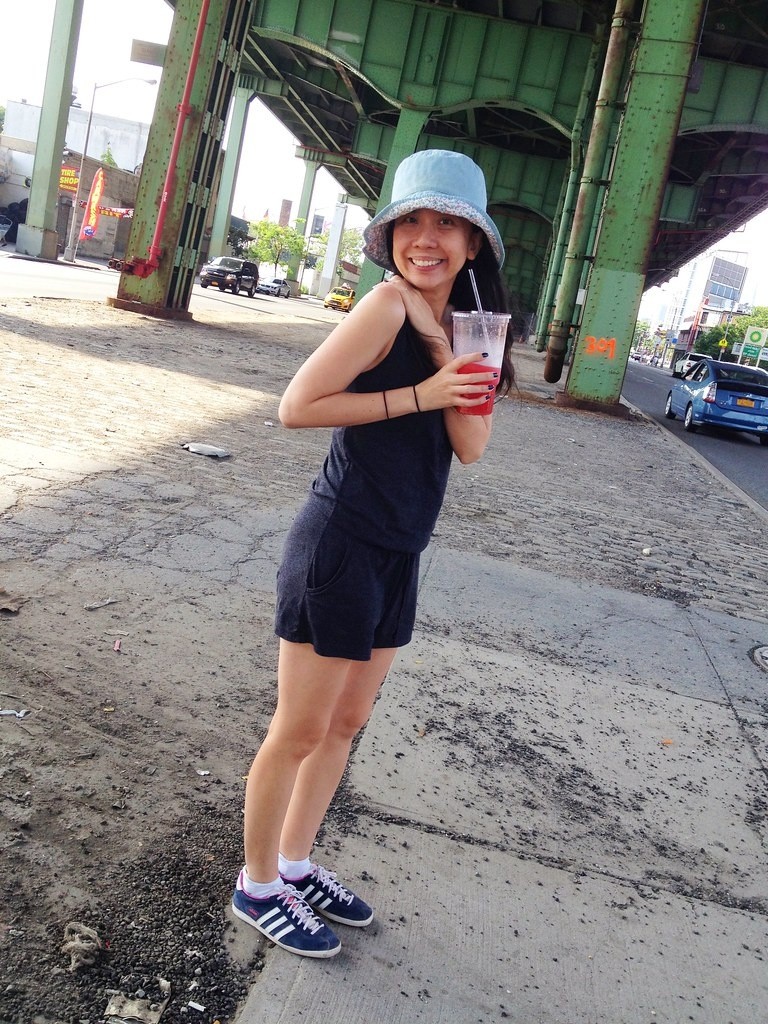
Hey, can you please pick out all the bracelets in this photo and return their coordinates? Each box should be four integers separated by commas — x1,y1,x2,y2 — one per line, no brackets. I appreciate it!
382,391,390,420
412,385,422,414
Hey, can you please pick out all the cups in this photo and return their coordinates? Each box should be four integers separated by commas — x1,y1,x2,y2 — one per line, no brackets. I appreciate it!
451,311,512,415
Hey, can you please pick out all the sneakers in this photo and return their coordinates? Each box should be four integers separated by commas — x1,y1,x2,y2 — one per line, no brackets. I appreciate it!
232,871,342,958
278,863,373,926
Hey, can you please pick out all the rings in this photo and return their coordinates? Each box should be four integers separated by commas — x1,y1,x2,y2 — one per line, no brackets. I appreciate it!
405,287,408,291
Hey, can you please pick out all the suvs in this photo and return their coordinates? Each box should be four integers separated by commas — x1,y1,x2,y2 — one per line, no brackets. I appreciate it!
671,351,713,380
198,255,259,298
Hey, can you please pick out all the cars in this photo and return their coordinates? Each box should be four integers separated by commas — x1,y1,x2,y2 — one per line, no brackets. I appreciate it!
255,279,292,299
664,358,768,445
323,282,356,313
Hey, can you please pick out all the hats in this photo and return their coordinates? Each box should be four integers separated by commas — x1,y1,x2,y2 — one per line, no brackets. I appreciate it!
362,149,505,274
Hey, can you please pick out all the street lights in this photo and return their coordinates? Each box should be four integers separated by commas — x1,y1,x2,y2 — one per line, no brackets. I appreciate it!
63,78,158,262
712,273,735,360
660,287,678,370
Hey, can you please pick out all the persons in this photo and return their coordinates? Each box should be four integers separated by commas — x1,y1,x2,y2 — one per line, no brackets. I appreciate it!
231,150,519,958
